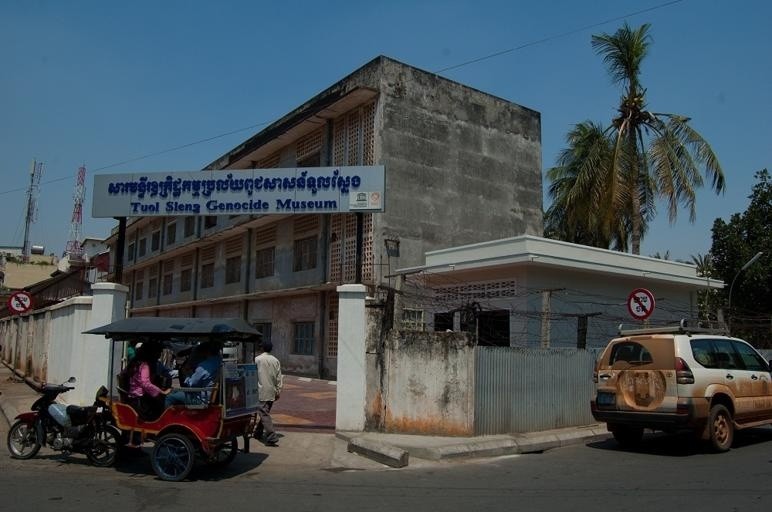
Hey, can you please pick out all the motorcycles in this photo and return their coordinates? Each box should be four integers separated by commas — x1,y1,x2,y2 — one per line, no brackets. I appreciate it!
6,316,263,482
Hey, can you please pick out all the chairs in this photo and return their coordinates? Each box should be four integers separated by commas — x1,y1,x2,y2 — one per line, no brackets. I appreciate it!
180,373,221,408
115,369,140,419
697,349,739,368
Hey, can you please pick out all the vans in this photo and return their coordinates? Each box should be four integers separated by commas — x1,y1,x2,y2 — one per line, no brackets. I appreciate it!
171,339,240,370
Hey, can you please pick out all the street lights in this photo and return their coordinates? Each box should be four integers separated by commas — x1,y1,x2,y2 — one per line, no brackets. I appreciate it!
728,252,763,330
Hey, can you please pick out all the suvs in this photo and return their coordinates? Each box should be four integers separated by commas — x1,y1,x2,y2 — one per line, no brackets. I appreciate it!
590,318,772,453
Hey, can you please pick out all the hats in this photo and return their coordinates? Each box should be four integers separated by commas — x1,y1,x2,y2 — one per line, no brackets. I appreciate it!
258,340,272,346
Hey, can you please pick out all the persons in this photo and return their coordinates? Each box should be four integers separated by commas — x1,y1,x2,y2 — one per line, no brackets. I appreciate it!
118,339,223,422
253,340,284,446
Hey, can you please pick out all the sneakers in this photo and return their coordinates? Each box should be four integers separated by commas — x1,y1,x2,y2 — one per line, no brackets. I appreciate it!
266,437,279,446
